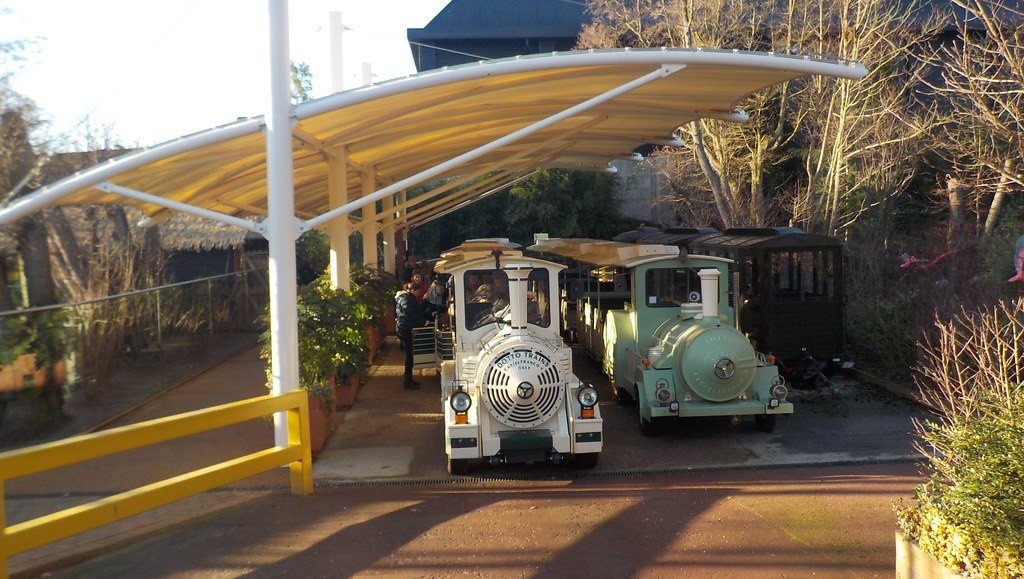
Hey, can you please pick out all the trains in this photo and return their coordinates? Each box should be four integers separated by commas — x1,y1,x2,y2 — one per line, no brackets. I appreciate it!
526,225,847,437
431,236,605,476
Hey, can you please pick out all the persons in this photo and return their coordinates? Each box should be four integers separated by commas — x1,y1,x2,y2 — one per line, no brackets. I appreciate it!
395,250,535,390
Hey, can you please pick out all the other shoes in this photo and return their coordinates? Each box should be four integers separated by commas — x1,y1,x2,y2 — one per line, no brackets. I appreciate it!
404,381,420,390
